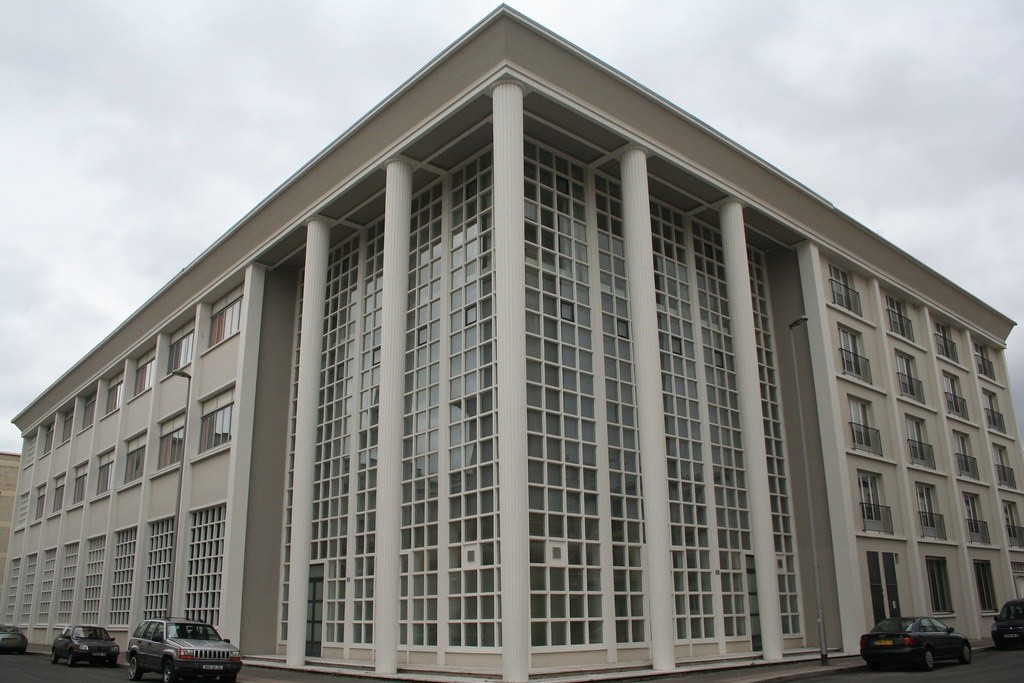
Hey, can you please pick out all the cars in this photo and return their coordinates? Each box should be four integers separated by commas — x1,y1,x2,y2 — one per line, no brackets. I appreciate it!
859,616,972,672
0,623,28,655
51,624,120,667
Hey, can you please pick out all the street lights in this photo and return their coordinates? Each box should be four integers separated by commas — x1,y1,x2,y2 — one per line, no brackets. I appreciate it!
788,315,830,665
166,369,191,618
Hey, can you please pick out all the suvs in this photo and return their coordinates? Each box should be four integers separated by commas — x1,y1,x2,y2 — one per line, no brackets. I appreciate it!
125,616,243,683
991,597,1024,650
